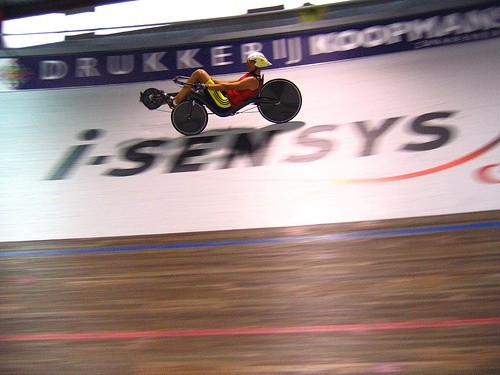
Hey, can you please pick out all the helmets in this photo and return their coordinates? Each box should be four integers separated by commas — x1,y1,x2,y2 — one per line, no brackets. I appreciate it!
247,51,272,69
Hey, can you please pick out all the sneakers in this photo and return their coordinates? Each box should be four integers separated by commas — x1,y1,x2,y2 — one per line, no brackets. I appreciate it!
160,90,178,110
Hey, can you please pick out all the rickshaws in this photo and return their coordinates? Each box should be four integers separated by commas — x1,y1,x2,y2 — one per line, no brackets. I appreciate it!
139,75,301,137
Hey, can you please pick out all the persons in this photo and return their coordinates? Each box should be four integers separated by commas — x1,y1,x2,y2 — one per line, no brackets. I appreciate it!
162,51,272,118
